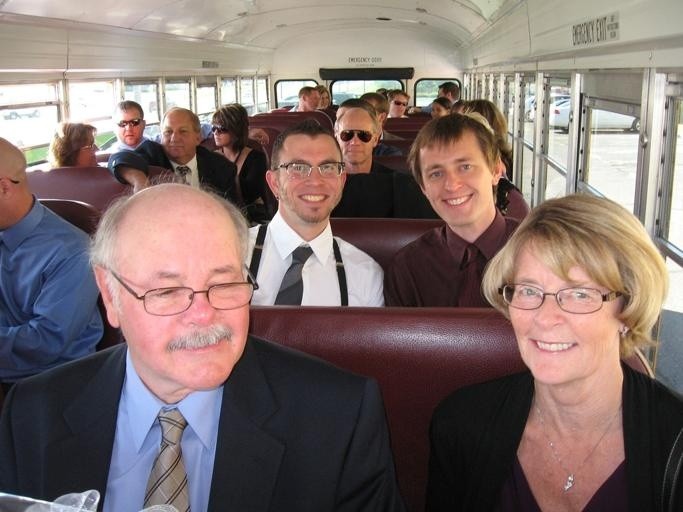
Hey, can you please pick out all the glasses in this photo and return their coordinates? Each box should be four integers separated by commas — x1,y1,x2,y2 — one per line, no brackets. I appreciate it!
275,162,346,180
81,140,94,150
108,263,259,317
498,283,622,314
212,125,228,134
340,129,372,143
395,101,406,107
117,118,140,128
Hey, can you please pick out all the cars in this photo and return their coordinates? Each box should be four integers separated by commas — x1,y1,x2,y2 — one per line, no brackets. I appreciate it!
519,85,653,146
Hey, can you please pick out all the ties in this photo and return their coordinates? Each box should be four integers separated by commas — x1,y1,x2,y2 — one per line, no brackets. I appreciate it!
143,407,192,512
456,246,483,307
274,246,312,306
177,166,189,185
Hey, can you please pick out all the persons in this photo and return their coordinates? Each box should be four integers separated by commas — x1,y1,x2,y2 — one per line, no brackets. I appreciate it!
1,134,104,414
426,81,513,184
334,87,417,192
50,100,271,223
2,183,405,512
384,113,525,311
288,85,332,113
423,197,683,512
235,122,385,309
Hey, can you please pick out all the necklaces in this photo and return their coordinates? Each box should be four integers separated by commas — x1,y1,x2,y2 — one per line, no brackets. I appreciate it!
532,393,622,493
